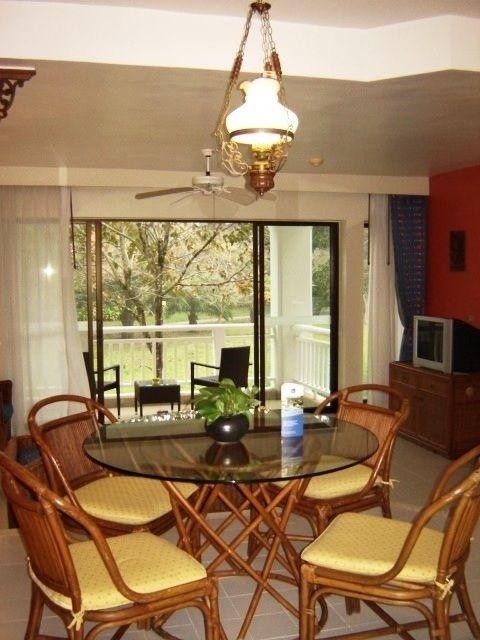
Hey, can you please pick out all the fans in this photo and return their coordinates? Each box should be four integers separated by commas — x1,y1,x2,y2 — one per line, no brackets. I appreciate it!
133,147,279,207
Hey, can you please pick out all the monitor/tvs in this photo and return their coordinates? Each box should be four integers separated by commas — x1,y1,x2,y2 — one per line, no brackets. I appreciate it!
412,316,480,375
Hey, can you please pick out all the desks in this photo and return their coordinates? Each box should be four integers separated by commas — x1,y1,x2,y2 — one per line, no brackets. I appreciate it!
134,379,181,416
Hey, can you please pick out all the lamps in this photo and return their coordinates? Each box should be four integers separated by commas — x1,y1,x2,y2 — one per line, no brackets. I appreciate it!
210,0,300,196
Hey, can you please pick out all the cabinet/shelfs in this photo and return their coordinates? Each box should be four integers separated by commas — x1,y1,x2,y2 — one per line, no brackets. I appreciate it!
387,360,480,461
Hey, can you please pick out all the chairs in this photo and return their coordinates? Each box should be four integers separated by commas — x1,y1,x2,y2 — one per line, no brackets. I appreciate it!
189,345,253,411
83,351,121,419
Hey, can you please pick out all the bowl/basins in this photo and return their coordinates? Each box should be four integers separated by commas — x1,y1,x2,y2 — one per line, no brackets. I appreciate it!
152,378,160,384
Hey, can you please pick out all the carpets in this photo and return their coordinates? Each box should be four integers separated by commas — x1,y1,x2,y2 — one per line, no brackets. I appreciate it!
0,423,337,538
